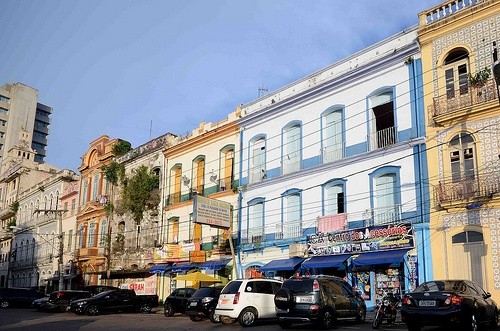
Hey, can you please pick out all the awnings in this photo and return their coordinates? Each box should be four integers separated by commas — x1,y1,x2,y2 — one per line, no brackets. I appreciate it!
170,261,201,272
353,248,413,267
301,254,352,274
199,260,234,270
148,263,172,274
259,259,305,275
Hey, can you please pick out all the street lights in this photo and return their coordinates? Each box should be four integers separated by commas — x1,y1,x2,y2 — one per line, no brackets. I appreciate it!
10,224,65,291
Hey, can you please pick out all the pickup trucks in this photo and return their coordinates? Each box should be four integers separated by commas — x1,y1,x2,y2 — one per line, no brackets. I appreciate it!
67,288,159,315
51,285,119,313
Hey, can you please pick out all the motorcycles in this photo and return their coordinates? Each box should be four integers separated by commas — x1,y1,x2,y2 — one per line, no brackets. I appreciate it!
370,290,399,328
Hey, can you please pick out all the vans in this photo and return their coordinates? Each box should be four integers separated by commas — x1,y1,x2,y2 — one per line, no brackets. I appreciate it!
0,287,49,309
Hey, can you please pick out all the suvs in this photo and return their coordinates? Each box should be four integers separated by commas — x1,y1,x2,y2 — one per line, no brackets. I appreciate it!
273,276,368,328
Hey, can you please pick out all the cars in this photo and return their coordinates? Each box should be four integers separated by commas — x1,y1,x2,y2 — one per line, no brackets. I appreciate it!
162,287,198,317
32,297,50,312
402,278,500,331
213,278,283,326
185,286,224,324
